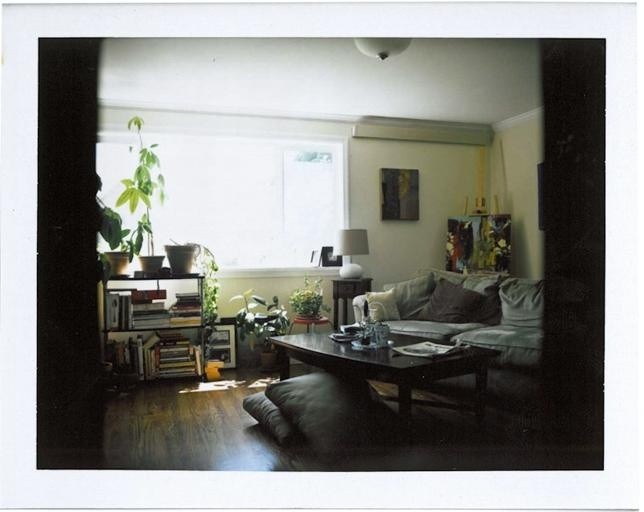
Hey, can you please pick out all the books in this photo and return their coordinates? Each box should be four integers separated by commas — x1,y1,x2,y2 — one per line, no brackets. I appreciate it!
329,321,362,344
170,293,202,325
105,293,132,330
131,289,171,328
106,332,204,381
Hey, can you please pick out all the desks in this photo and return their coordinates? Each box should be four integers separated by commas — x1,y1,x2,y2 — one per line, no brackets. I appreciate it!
332,278,372,331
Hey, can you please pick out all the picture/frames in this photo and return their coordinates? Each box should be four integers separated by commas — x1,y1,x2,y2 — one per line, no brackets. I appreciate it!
311,250,321,266
322,246,343,266
204,323,237,370
379,168,419,221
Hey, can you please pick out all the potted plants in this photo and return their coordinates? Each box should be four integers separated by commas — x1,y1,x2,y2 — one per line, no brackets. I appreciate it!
229,289,290,368
103,221,152,275
289,274,331,320
165,241,218,337
119,114,165,274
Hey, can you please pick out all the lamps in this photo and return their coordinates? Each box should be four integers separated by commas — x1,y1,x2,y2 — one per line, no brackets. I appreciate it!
332,229,370,279
354,38,411,61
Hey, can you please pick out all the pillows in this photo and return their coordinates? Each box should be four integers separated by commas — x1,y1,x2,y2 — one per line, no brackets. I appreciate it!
416,278,481,325
365,287,401,322
384,273,435,320
265,367,400,464
243,390,294,444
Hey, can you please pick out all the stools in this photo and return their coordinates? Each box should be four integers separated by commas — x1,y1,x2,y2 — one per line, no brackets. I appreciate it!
289,317,335,334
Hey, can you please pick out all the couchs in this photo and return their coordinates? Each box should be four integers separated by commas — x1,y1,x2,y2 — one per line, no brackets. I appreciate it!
352,267,547,370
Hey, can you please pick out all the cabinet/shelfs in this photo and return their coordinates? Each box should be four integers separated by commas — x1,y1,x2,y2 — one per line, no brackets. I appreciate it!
101,274,207,383
